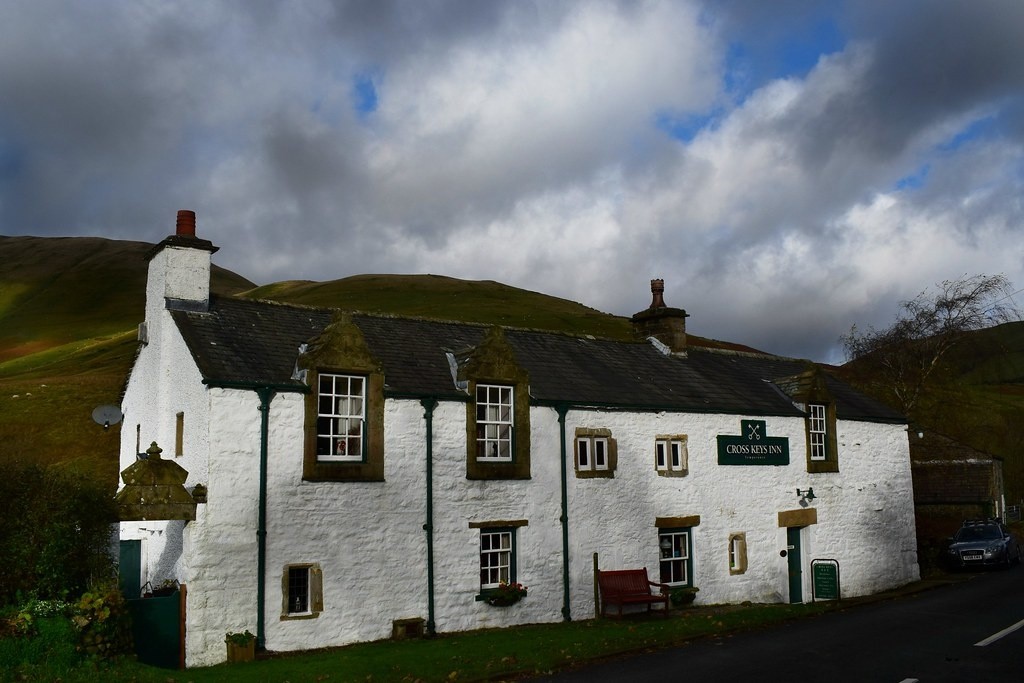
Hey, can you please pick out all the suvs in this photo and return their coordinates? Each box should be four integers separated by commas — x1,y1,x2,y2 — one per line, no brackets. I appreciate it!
946,518,1022,571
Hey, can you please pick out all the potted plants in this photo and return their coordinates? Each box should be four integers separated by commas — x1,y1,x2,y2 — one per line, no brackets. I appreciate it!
225,631,255,663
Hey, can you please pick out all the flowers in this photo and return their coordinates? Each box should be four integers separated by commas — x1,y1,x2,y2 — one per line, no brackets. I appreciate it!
499,580,524,594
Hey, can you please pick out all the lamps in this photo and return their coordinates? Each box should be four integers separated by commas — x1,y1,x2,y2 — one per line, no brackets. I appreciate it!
798,488,816,501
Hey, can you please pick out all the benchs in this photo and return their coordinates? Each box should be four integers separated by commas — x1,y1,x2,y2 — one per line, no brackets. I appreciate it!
596,567,669,617
